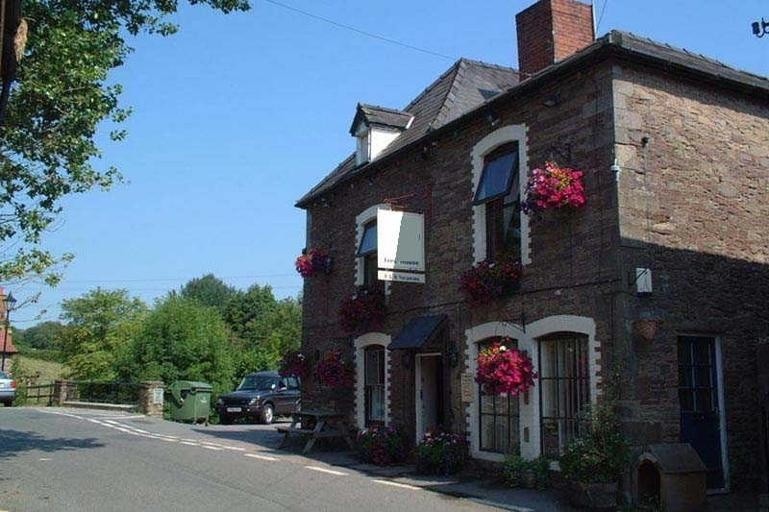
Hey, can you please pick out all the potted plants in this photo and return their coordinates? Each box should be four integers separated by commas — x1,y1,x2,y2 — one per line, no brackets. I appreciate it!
502,454,550,492
558,436,619,511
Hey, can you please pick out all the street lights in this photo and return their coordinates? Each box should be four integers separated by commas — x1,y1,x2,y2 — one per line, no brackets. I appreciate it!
2,291,17,371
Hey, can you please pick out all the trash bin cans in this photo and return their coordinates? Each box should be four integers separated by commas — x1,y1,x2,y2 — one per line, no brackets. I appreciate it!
167,381,213,425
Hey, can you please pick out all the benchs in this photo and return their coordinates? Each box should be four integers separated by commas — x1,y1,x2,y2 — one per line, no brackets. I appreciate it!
274,409,355,456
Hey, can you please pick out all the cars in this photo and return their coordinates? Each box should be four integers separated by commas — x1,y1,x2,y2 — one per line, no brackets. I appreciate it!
0,370,20,406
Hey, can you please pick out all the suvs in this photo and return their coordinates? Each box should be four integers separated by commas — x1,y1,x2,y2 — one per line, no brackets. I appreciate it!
215,370,302,424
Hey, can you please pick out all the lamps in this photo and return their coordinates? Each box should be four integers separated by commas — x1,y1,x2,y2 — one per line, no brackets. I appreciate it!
322,254,332,275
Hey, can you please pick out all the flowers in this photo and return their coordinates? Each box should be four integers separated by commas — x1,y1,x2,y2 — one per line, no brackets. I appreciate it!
457,258,522,311
476,338,537,399
312,351,356,390
278,351,310,380
295,247,318,276
339,286,385,337
356,424,407,467
417,432,472,475
523,159,584,221
